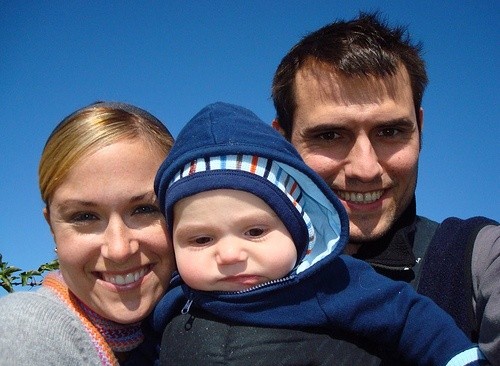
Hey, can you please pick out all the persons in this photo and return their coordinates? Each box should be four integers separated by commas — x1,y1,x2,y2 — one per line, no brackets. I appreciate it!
0,100,176,366
142,101,491,366
271,9,500,366
